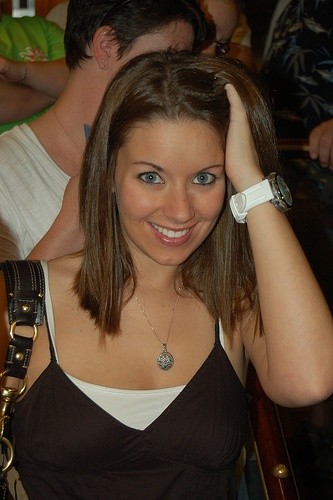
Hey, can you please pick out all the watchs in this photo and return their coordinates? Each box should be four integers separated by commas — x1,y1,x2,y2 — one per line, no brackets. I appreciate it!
229,172,292,223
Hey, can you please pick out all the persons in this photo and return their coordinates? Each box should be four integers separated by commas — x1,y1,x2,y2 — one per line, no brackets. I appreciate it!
0,1,333,500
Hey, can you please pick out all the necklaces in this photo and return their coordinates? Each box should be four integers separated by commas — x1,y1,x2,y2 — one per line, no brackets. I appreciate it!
125,284,185,370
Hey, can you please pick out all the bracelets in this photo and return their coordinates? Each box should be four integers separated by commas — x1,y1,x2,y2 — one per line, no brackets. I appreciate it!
18,63,28,83
